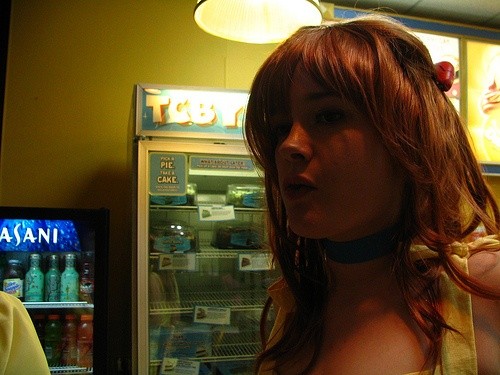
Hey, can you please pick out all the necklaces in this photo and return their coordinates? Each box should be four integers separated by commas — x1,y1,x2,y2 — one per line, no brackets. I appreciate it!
323,227,400,264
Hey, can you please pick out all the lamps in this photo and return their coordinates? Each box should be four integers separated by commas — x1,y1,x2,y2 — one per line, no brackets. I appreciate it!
193,0,324,43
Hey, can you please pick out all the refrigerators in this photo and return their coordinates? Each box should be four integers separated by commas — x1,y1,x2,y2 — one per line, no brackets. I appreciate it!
0,205,138,375
133,82,287,375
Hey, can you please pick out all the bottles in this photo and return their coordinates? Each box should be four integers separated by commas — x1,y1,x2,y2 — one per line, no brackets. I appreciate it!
35,315,93,367
4,253,95,304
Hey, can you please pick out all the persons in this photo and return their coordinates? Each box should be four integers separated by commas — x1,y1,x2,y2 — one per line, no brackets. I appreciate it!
241,14,500,375
